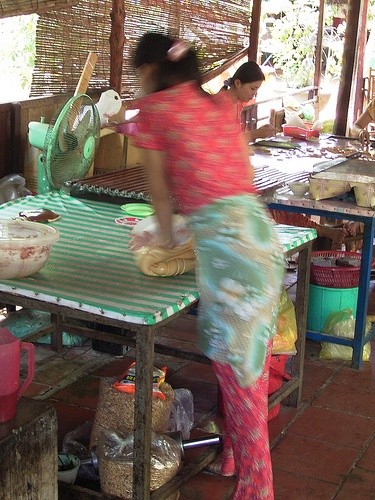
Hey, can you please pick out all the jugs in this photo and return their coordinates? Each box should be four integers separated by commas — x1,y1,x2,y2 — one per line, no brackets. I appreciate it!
0,329,35,423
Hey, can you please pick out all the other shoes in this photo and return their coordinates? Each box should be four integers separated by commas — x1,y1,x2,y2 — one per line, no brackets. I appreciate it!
201,460,237,478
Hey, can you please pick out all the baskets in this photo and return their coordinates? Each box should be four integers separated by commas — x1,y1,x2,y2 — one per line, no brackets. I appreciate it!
281,121,321,140
295,250,375,287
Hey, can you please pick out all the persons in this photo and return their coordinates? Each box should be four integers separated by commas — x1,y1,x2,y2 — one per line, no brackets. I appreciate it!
342,99,375,236
219,61,347,243
131,32,286,500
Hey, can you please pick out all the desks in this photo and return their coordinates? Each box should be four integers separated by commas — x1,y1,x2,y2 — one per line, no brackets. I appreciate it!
0,189,318,500
265,158,375,370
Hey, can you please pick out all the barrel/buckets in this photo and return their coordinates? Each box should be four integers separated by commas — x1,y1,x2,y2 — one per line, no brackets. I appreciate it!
306,283,359,332
268,354,292,423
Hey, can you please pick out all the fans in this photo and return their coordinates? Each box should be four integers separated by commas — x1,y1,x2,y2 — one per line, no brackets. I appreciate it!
28,94,102,194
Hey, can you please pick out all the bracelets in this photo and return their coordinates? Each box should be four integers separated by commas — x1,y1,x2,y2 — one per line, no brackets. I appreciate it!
358,129,363,137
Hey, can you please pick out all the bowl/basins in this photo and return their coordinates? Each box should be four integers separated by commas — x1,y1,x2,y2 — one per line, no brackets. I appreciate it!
58,452,80,484
0,218,60,280
288,183,309,196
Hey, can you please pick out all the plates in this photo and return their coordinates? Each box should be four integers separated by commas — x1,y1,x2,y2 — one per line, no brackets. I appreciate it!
121,203,156,218
114,217,144,226
